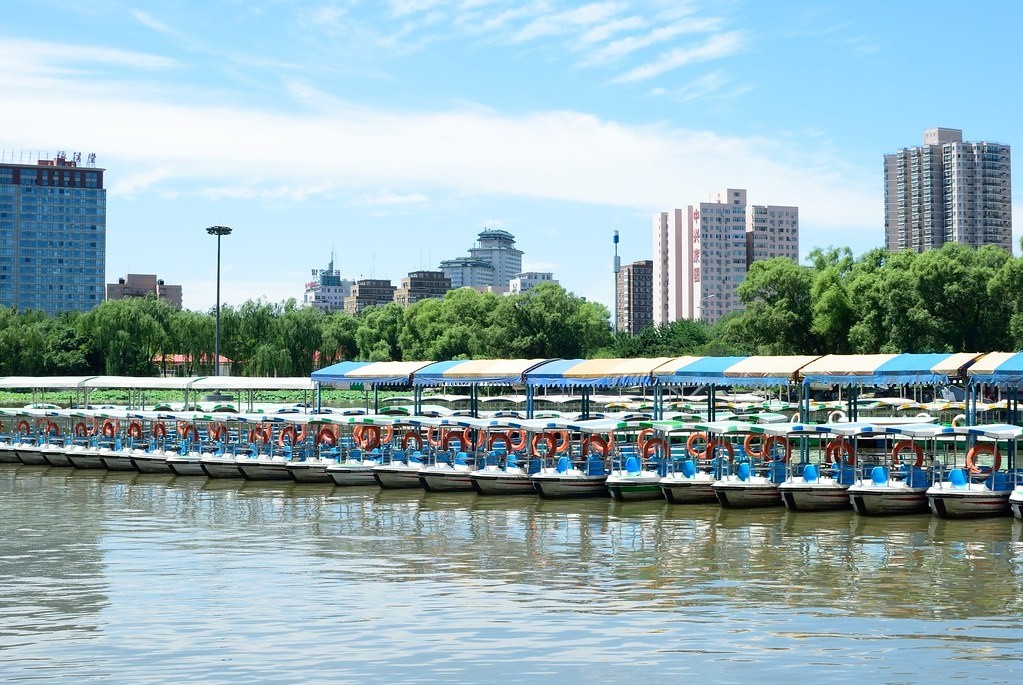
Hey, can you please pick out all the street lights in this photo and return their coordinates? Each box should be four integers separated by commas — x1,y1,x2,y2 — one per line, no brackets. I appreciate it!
205,225,235,395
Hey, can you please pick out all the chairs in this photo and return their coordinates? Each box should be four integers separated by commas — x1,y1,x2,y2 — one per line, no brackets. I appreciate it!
0,431,1023,490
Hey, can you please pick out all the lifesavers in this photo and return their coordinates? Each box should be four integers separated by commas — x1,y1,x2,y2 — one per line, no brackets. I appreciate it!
966,444,1001,473
402,432,422,450
952,413,966,427
488,430,526,460
17,420,30,435
36,418,59,436
248,424,271,445
686,433,734,462
790,413,801,423
638,428,671,458
315,424,339,444
826,436,855,466
531,431,569,458
427,428,486,452
153,421,166,439
916,412,934,424
828,410,845,424
208,422,229,442
127,420,142,439
278,424,306,446
102,418,120,437
583,432,613,460
353,424,392,450
76,418,98,436
744,433,791,463
178,421,198,442
892,441,924,467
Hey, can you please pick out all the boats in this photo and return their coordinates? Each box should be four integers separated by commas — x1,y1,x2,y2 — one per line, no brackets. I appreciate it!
0,408,967,515
1007,483,1023,519
924,422,1023,517
